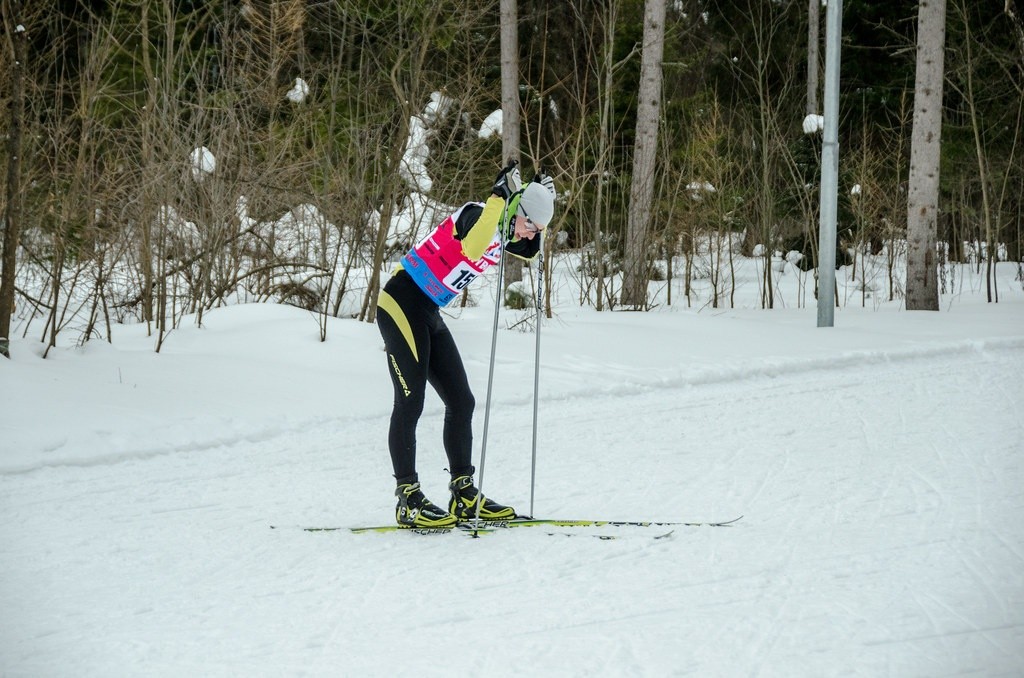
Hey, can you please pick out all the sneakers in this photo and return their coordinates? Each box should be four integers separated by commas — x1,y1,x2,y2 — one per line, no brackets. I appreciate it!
394,482,458,527
448,475,517,520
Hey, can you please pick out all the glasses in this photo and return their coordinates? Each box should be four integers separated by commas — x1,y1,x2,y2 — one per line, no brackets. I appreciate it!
519,202,542,234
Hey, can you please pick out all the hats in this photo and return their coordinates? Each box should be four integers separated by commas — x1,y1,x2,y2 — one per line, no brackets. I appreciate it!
504,182,554,227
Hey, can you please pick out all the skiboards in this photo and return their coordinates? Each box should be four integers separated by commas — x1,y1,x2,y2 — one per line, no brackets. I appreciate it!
270,515,746,541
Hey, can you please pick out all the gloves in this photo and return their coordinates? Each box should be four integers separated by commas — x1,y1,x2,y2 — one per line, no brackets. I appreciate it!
534,174,556,199
492,167,521,200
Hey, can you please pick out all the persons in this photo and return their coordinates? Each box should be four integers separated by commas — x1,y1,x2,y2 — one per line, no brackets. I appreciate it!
376,166,556,530
